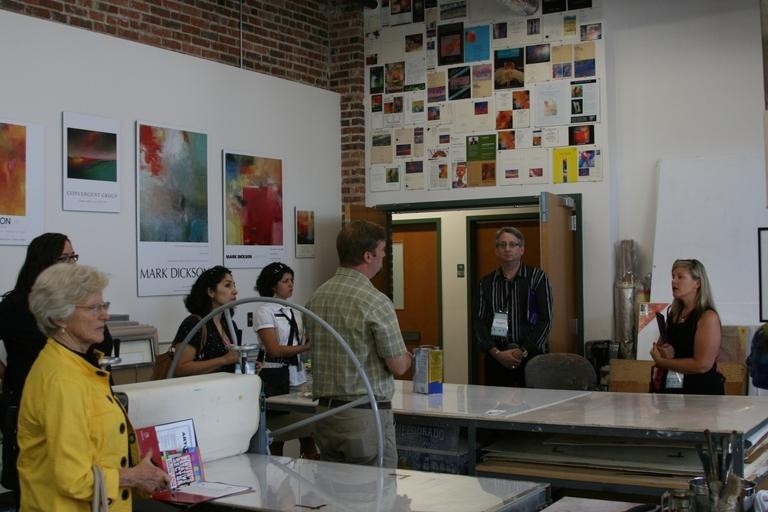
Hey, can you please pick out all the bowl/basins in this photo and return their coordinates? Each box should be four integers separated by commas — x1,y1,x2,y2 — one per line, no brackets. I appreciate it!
687,476,758,512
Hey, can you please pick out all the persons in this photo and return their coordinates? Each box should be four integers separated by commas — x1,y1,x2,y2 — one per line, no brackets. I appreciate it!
301,221,414,469
253,261,310,457
172,266,242,376
0,233,114,493
15,263,170,512
650,259,726,395
469,227,553,386
745,324,768,390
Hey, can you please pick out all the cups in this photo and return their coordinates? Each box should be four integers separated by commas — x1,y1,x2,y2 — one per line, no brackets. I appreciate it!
661,488,698,512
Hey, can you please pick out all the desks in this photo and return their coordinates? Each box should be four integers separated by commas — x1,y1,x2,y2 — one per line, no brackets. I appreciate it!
129,453,552,512
262,378,768,498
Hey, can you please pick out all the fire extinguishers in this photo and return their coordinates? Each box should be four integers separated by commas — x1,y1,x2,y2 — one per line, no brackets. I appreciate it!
635,279,650,331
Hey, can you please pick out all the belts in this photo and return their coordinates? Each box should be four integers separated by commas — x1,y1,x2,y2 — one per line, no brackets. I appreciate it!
318,397,392,409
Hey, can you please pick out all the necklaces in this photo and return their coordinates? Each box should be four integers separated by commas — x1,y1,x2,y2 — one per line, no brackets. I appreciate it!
679,310,689,322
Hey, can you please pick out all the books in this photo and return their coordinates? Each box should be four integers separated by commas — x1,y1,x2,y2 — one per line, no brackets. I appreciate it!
134,418,255,509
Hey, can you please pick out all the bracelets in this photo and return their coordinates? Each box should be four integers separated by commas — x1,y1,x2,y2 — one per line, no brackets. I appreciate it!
492,350,500,357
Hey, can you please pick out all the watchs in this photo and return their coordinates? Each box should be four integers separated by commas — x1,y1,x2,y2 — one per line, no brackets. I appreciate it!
519,347,528,358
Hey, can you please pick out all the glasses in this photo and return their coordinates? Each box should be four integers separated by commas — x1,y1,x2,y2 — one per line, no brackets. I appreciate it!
59,255,79,264
499,241,519,247
273,265,283,274
75,302,110,313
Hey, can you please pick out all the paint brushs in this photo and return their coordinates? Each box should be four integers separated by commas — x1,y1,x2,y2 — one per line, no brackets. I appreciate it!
709,481,723,512
696,429,744,485
720,473,741,512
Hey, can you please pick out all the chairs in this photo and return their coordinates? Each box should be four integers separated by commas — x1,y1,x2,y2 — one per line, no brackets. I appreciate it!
524,352,598,391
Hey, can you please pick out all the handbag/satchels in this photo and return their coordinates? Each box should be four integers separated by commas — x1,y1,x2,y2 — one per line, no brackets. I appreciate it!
255,365,289,395
154,314,208,380
649,364,664,393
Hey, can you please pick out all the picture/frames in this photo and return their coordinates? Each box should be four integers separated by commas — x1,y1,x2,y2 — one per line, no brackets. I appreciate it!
758,227,768,323
